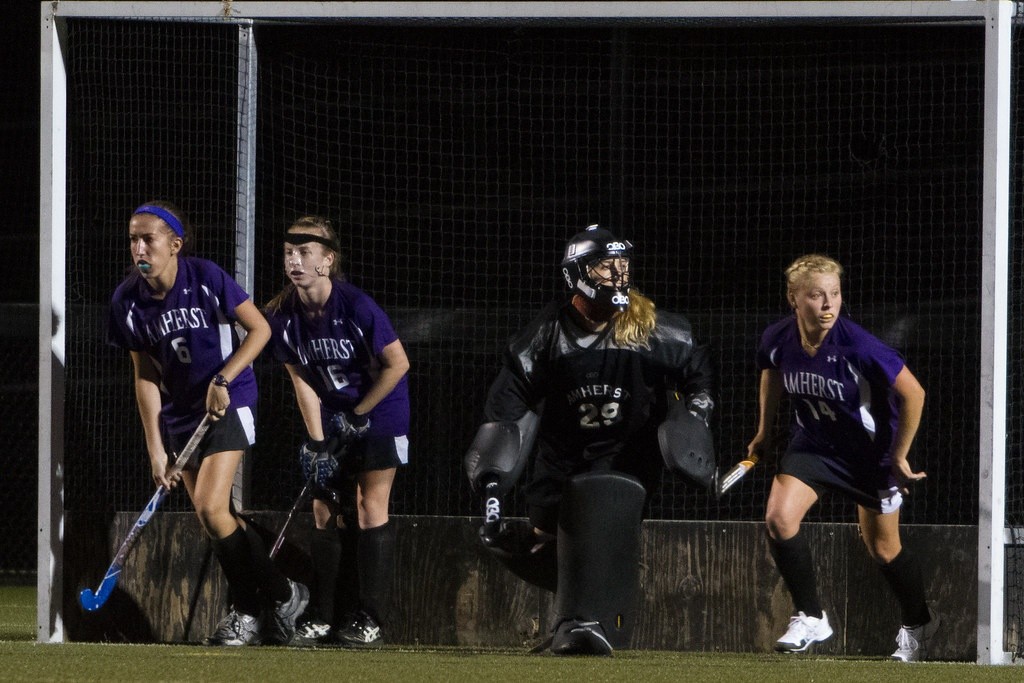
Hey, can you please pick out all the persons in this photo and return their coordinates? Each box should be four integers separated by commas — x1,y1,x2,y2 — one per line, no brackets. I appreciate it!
259,216,411,647
466,223,714,655
109,201,309,647
748,254,943,662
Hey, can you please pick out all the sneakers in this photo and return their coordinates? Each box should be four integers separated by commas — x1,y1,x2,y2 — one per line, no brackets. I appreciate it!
550,619,613,656
269,577,310,646
336,611,384,645
214,610,263,646
295,620,331,640
771,609,833,654
889,628,920,661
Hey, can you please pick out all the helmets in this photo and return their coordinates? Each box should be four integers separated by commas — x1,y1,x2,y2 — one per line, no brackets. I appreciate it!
559,223,634,313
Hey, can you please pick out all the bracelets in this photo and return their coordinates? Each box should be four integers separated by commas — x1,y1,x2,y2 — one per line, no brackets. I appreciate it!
210,375,228,386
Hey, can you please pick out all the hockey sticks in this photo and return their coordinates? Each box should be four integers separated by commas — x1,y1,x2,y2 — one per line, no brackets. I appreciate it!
713,444,766,505
269,431,338,565
482,474,532,548
78,391,231,611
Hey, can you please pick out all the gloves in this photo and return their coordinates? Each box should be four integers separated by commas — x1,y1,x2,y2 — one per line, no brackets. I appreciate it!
297,441,341,499
324,407,373,443
